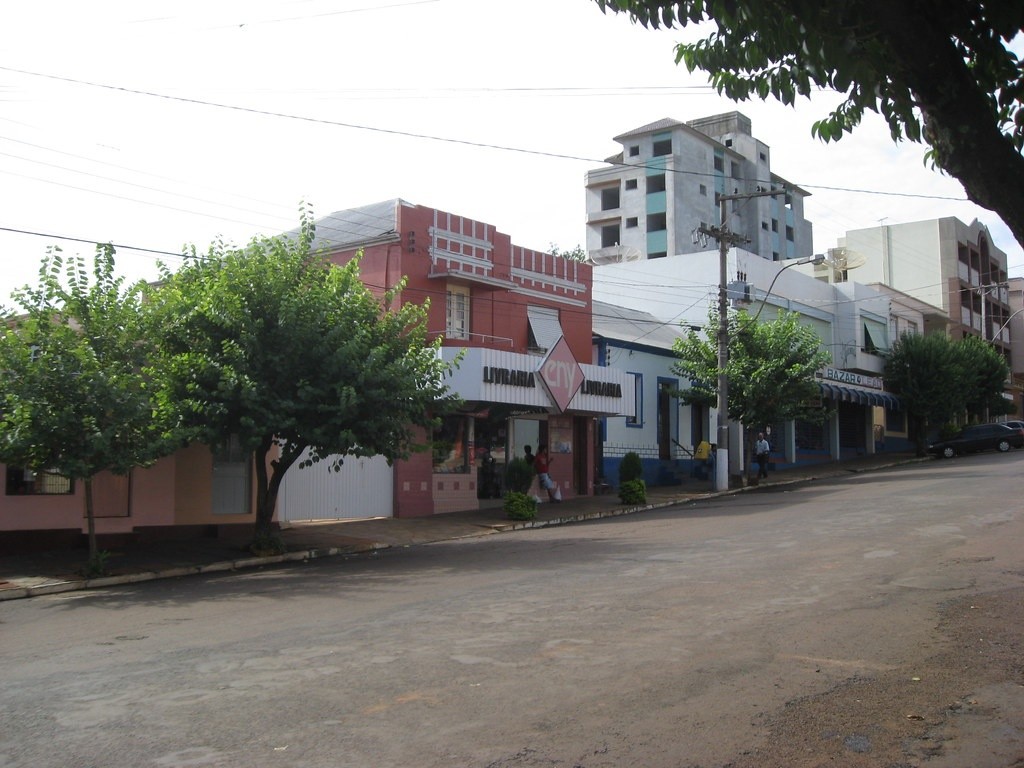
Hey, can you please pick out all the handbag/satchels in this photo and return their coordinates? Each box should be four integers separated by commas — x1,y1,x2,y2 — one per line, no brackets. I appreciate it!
762,451,769,463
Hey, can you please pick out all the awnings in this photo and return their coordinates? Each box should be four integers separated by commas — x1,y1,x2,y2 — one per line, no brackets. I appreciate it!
527,305,564,349
860,315,890,355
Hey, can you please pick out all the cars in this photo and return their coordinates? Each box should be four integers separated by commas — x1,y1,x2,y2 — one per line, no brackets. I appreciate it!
926,423,1024,459
1001,420,1024,449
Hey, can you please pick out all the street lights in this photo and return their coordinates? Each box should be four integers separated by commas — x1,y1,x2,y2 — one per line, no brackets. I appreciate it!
715,253,827,493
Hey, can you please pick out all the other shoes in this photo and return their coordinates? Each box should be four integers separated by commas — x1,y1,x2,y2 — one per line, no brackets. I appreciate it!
550,498,560,502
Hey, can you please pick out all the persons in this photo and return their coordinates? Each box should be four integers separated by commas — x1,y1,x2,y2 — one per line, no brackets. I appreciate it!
755,432,770,479
533,445,561,502
709,444,717,458
